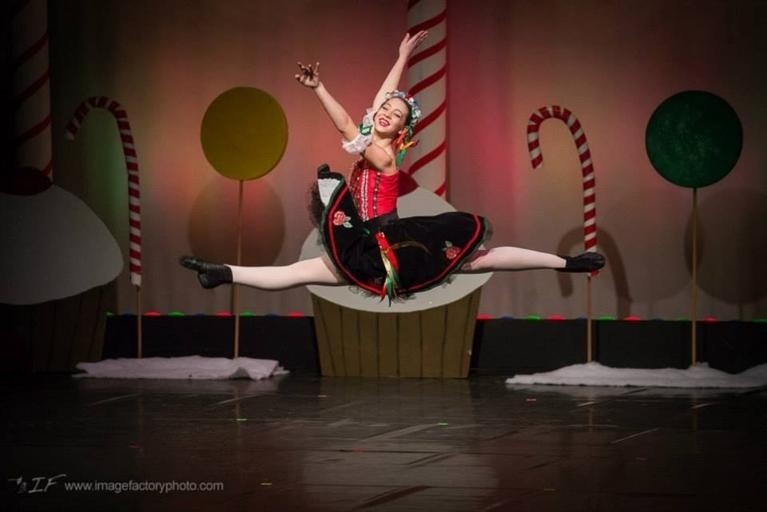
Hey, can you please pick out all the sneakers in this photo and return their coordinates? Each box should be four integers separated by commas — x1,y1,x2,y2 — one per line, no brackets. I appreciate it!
179,257,232,288
558,253,604,273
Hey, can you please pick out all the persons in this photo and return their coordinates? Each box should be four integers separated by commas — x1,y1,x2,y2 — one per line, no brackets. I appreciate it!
175,27,608,308
